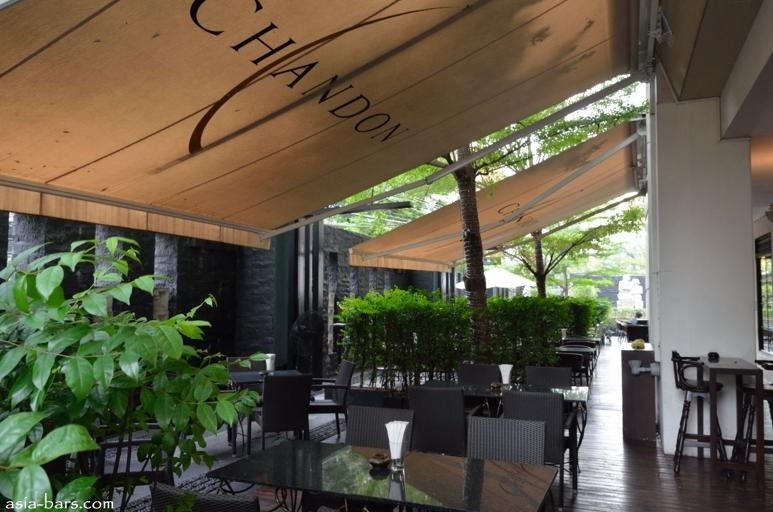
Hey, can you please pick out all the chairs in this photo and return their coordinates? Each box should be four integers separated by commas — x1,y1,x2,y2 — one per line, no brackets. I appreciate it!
99,317,772,512
